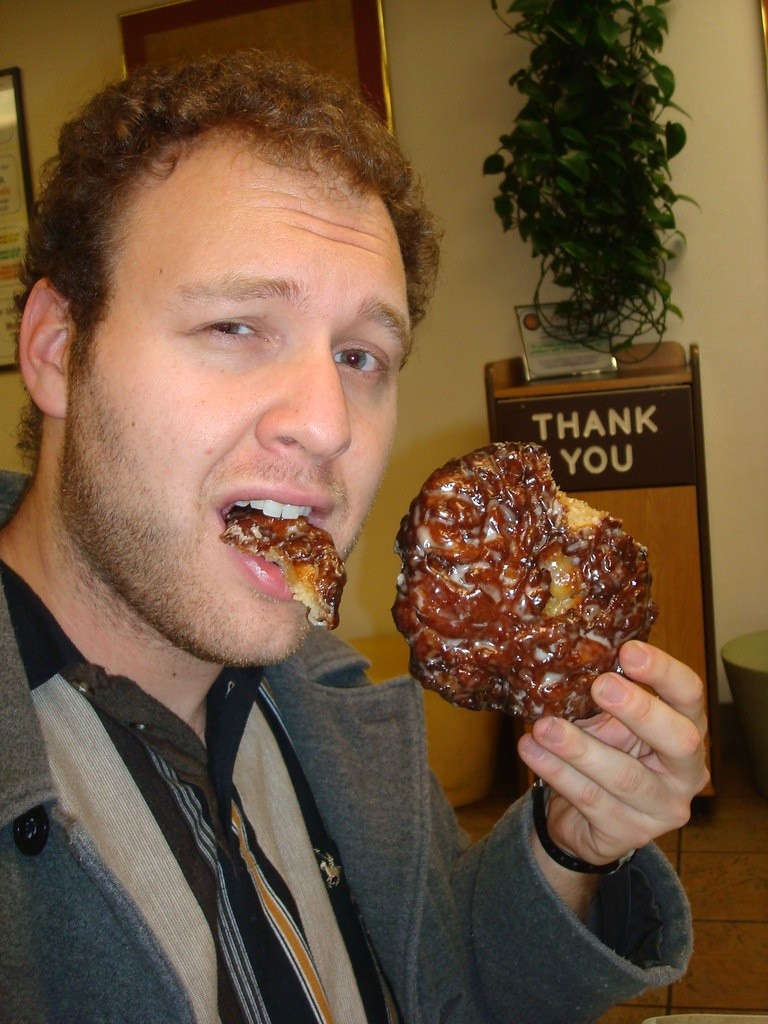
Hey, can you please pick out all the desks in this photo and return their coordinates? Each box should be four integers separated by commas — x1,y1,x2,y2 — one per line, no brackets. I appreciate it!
486,343,716,817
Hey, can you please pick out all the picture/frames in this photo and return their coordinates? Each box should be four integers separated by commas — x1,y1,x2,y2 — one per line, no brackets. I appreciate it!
118,0,394,143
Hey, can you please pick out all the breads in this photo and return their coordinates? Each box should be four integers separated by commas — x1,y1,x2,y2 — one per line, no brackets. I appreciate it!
384,436,654,722
218,509,348,631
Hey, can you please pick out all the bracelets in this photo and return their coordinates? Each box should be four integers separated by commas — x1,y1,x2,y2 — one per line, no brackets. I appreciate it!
531,775,636,881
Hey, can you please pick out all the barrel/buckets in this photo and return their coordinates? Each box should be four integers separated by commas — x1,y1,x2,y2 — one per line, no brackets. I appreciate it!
347,635,501,810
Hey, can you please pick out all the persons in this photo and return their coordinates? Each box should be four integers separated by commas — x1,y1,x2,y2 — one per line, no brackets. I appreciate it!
1,44,714,1024
